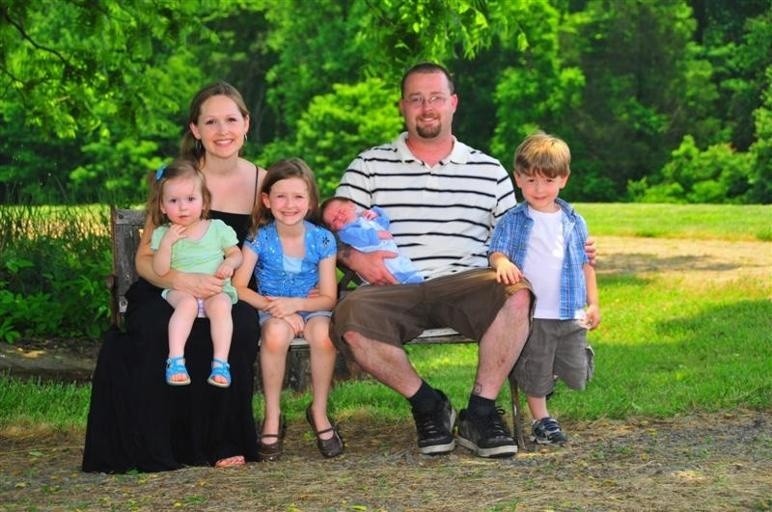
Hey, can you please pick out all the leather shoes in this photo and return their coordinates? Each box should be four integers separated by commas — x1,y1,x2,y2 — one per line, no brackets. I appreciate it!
305,400,345,459
257,410,288,461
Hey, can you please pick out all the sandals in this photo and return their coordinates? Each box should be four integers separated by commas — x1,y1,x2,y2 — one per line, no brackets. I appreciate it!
165,355,192,386
207,358,232,388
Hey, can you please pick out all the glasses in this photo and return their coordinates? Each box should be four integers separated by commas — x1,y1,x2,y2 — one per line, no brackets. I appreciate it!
405,94,450,105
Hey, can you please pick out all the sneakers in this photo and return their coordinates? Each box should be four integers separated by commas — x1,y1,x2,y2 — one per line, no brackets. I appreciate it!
529,417,567,444
411,388,458,454
456,408,518,458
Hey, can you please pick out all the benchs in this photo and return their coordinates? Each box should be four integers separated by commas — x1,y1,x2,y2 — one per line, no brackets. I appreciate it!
110,204,529,452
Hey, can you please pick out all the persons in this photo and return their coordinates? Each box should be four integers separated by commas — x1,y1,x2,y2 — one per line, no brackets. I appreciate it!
145,160,243,388
320,196,425,284
331,63,600,457
232,158,343,458
489,133,601,445
78,81,270,474
